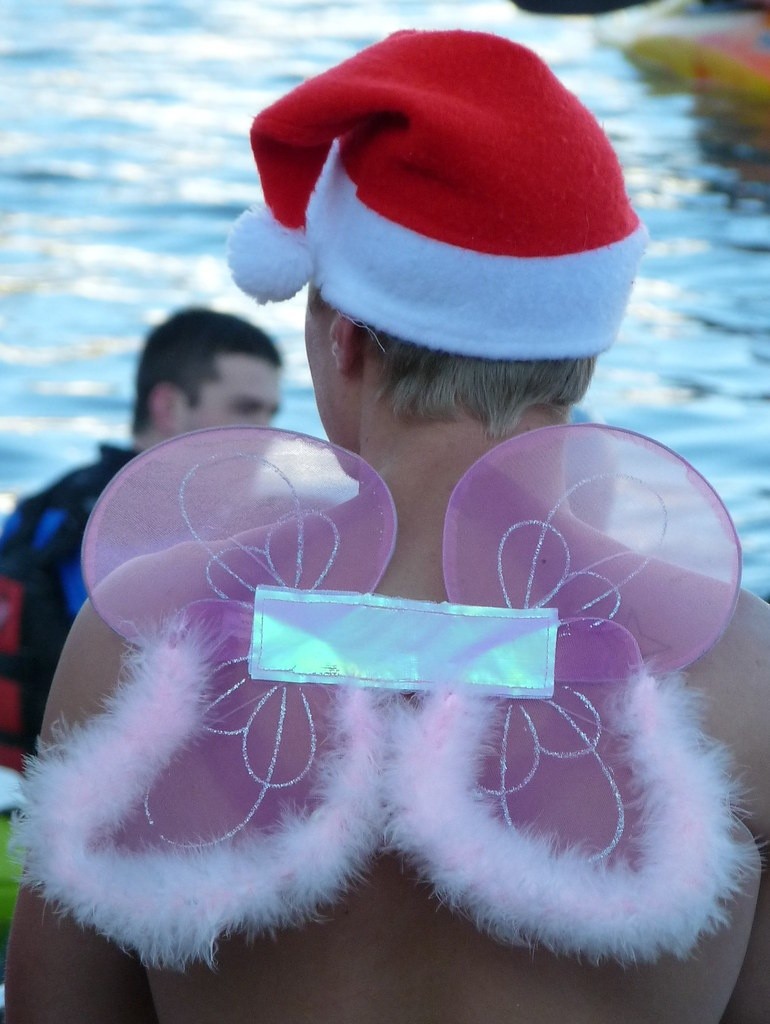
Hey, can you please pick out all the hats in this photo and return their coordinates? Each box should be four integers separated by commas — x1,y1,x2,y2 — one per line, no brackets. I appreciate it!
227,30,650,361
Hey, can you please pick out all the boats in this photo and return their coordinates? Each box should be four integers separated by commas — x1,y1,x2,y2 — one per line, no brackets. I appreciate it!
578,0,770,104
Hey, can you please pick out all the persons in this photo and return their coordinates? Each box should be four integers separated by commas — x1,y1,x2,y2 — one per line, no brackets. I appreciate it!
0,30,769,1024
0,305,285,819
